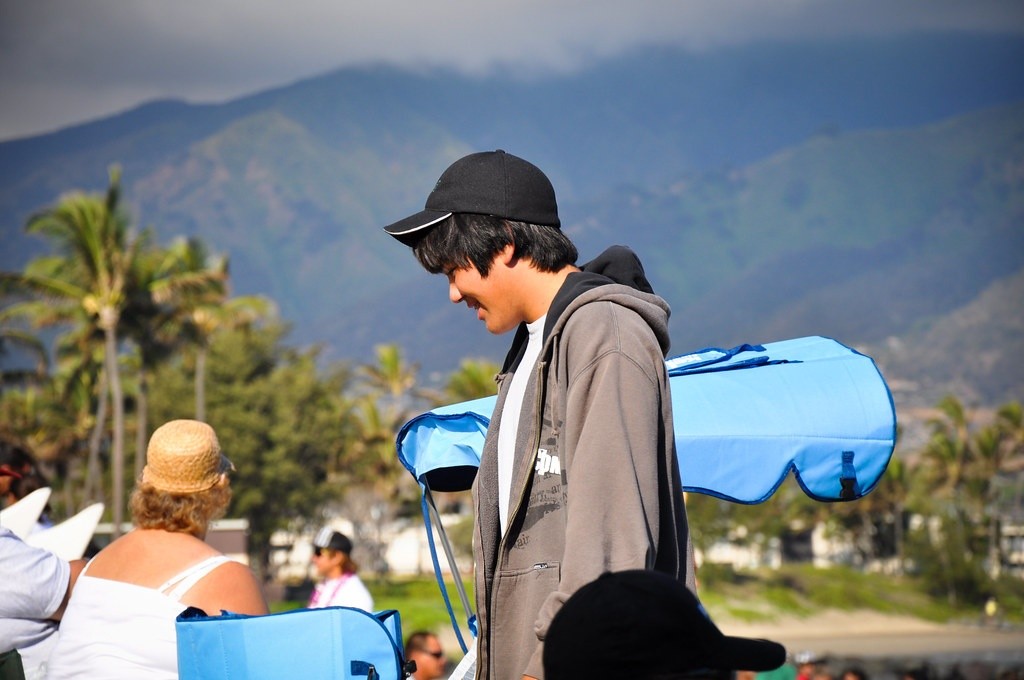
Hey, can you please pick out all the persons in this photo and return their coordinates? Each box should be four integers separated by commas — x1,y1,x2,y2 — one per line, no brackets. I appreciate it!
404,633,447,680
42,419,270,680
381,149,691,680
543,570,1024,680
307,528,374,614
0,445,90,680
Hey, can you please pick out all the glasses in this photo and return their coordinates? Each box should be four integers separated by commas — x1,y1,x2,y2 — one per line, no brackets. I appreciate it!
417,646,442,659
1,466,23,480
315,546,322,556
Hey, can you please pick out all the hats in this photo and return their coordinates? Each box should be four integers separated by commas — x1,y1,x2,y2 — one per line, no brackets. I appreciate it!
141,420,227,493
382,149,562,236
541,571,787,679
313,517,354,554
793,647,827,664
